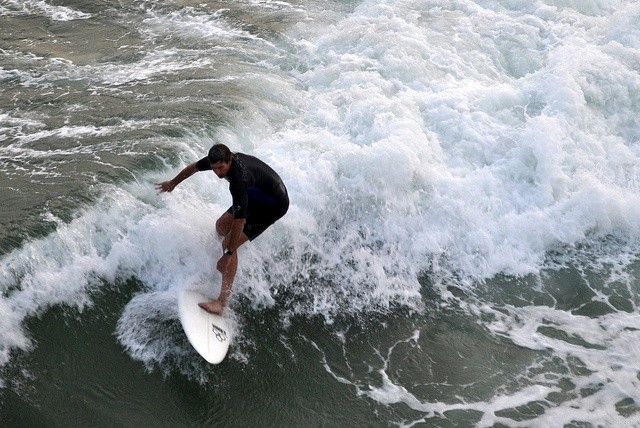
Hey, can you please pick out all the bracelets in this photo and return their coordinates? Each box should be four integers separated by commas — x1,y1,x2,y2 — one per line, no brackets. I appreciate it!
224,247,232,254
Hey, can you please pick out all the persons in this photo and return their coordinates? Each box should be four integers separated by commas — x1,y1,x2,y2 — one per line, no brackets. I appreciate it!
155,142,290,316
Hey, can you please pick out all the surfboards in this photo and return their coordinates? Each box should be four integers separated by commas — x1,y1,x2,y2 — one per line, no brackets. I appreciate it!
178,291,231,365
